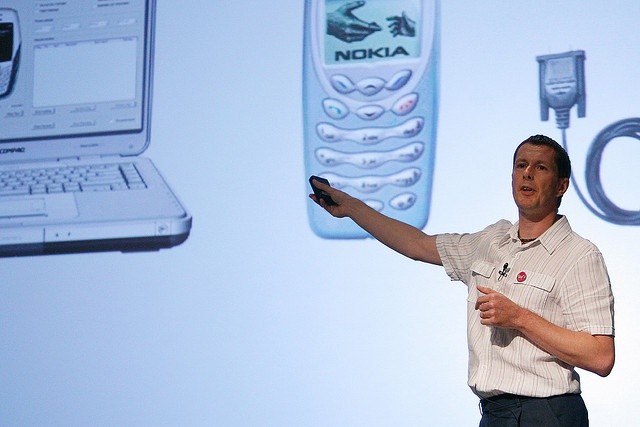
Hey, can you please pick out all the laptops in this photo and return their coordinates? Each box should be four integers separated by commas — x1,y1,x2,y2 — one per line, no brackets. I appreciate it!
0,1,193,257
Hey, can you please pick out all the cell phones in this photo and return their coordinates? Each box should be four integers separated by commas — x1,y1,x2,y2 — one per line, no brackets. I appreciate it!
303,0,440,239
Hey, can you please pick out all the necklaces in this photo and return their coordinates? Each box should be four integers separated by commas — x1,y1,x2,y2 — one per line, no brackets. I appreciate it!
518,230,537,244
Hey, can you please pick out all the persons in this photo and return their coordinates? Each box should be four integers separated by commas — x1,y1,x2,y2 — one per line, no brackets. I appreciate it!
326,1,381,42
309,135,615,427
386,11,415,37
